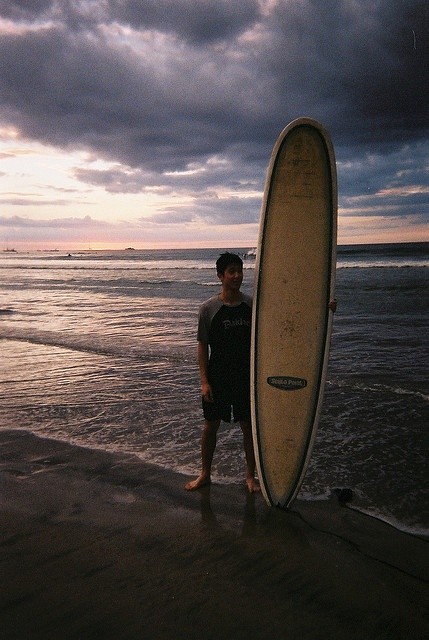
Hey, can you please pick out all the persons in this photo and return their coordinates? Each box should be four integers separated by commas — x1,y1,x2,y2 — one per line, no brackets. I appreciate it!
184,251,339,497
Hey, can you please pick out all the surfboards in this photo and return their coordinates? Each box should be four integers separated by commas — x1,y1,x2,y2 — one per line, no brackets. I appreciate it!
250,116,337,508
